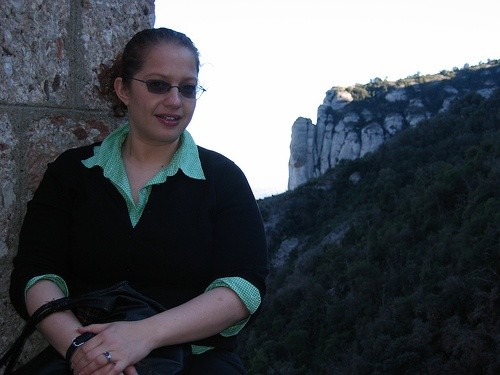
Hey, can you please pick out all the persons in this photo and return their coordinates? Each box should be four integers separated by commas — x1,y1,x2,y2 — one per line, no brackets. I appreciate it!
9,27,266,375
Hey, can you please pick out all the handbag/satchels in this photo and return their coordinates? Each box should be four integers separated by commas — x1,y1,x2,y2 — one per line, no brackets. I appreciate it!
0,280,193,375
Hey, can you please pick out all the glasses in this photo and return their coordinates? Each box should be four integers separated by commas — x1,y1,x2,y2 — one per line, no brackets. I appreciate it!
123,76,206,99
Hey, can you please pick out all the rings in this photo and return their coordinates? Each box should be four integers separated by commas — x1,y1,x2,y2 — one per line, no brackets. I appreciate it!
104,351,112,364
